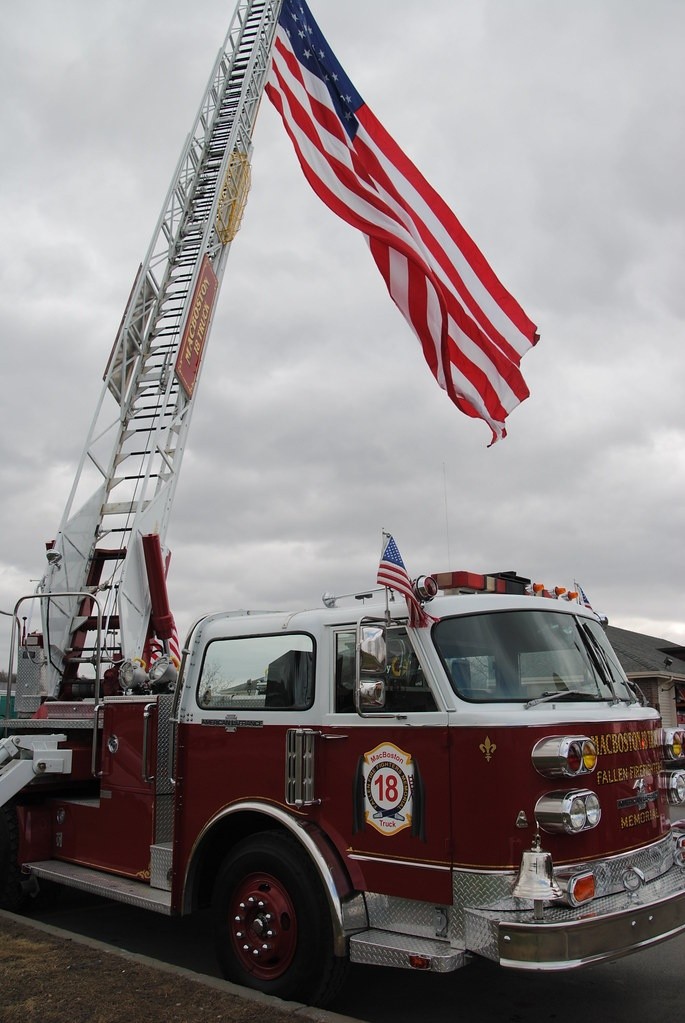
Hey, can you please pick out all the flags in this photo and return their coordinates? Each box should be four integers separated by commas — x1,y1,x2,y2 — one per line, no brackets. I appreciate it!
575,586,592,610
265,0,539,446
148,617,179,669
379,537,439,629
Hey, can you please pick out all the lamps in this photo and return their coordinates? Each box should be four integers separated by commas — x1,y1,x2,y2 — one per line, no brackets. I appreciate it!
663,656,674,666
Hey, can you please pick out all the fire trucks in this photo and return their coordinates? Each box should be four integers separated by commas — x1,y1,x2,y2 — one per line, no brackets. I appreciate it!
1,0,685,1011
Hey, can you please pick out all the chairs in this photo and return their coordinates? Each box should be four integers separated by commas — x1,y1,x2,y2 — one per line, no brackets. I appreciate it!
452,657,472,697
